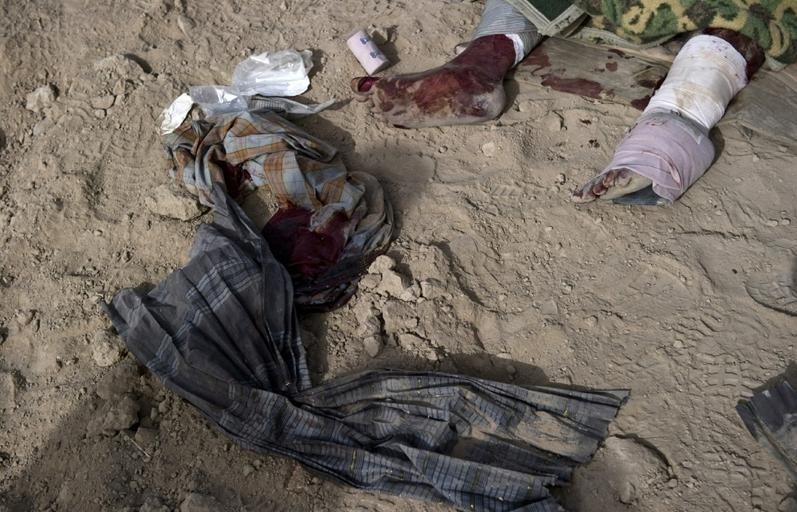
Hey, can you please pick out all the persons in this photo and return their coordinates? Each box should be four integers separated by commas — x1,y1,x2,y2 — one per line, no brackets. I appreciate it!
348,1,796,204
